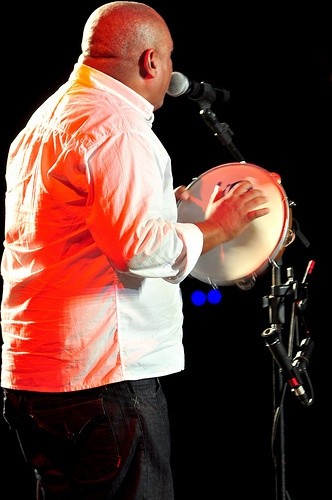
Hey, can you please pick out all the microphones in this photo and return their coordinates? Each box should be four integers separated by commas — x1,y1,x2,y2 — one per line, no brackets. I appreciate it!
262,328,309,406
167,72,230,102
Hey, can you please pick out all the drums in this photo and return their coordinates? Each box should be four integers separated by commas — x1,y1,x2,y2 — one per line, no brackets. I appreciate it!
174,163,297,290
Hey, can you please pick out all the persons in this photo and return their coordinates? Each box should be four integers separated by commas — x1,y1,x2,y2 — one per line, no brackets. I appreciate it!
1,1,270,500
